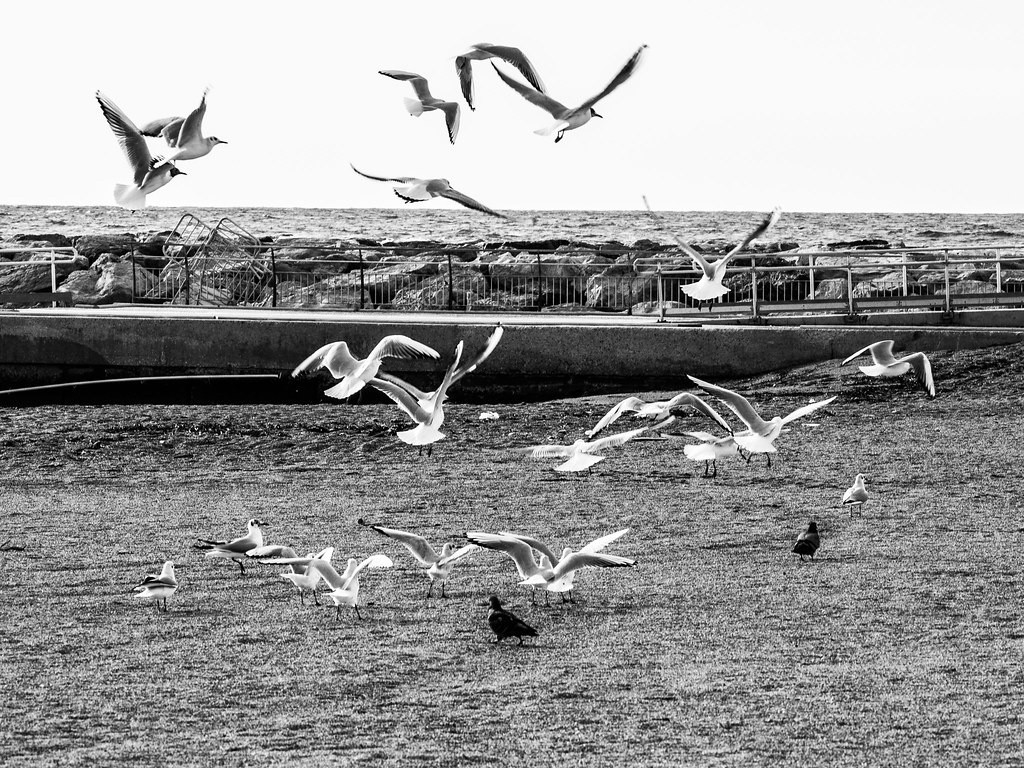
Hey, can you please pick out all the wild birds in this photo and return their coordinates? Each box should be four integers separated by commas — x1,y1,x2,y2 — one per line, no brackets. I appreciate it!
349,161,510,223
484,43,648,144
377,69,461,146
290,322,506,447
357,516,637,648
642,193,783,303
840,338,937,400
245,541,394,622
93,89,230,208
192,518,270,577
131,560,180,613
455,41,548,113
841,472,871,521
792,521,822,566
506,374,839,482
